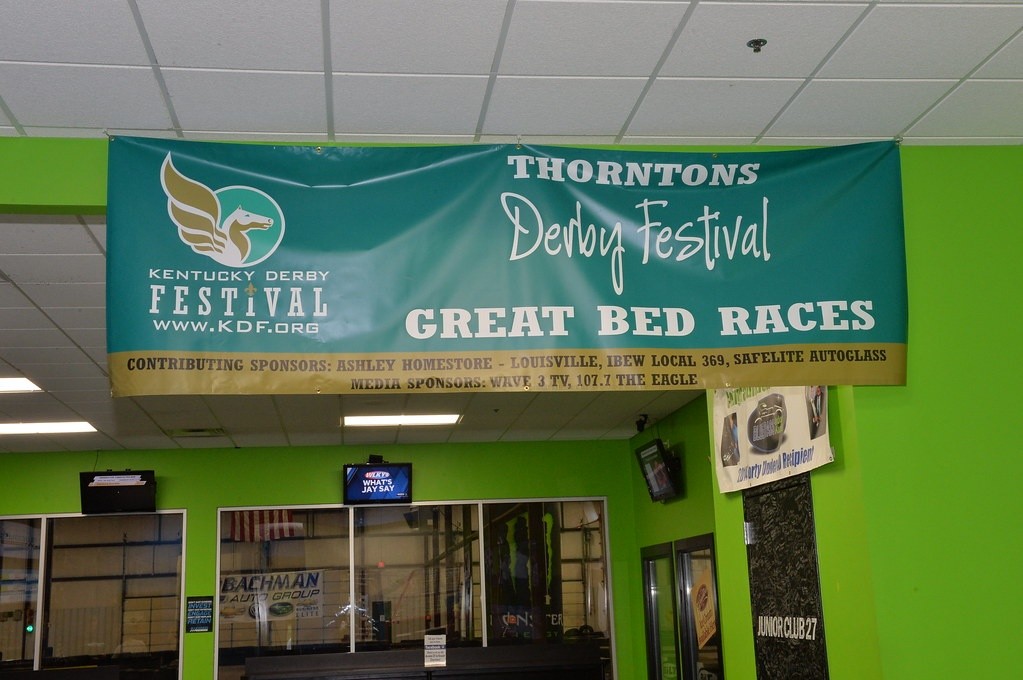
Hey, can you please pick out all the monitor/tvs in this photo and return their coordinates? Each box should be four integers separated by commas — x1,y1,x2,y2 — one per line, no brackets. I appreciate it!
634,438,678,503
79,470,157,515
343,462,412,504
